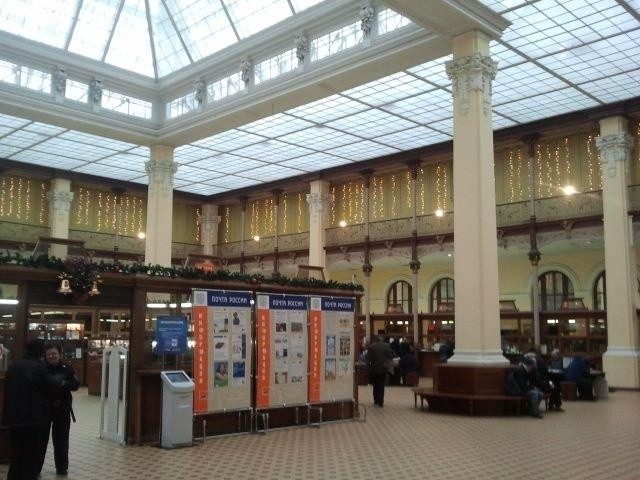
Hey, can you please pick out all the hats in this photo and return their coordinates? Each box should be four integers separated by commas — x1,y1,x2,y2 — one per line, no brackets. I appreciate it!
27,339,44,348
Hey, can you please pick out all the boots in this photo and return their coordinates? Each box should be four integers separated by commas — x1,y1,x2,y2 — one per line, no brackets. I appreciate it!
533,402,564,417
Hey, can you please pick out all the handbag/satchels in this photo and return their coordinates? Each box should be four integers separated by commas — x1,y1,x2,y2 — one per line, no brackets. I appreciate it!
507,374,522,396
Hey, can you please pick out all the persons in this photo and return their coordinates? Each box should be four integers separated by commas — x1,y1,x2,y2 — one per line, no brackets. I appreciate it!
438,340,454,362
42,344,81,475
506,346,565,418
215,363,227,379
365,331,417,407
4,339,64,480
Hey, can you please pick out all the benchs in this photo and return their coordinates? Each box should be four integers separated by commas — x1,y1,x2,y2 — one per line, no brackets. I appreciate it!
410,386,562,417
